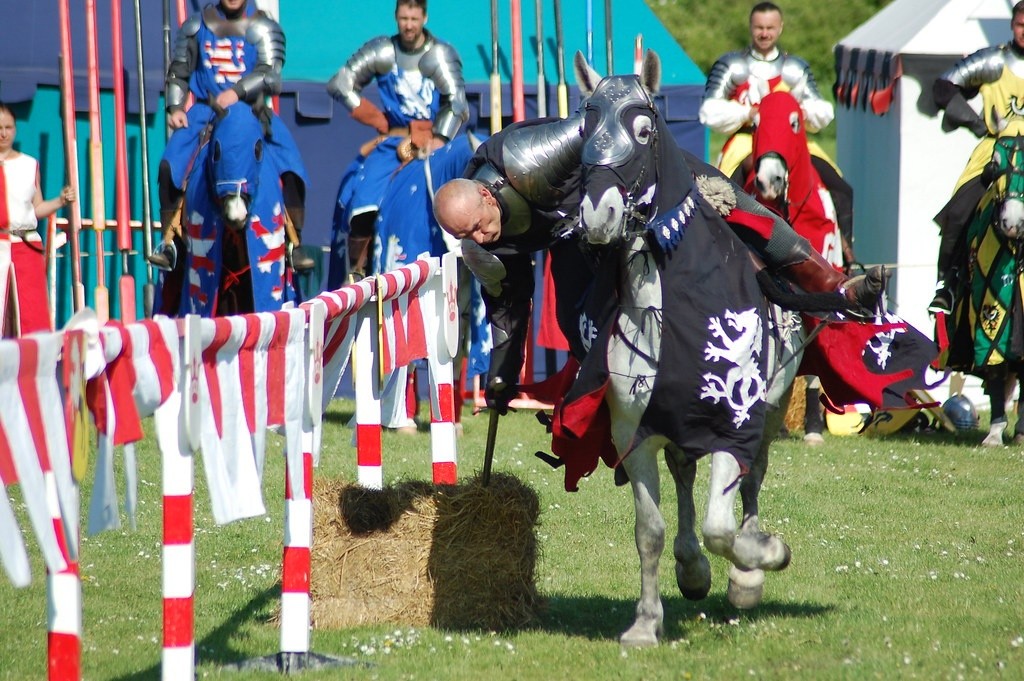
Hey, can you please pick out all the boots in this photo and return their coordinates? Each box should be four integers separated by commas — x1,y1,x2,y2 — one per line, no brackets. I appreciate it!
343,234,372,286
775,241,886,310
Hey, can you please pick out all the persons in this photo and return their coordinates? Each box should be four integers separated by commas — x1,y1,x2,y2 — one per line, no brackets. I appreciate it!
327,0,469,292
699,3,853,277
0,101,76,341
146,0,315,272
927,4,1024,316
432,105,887,415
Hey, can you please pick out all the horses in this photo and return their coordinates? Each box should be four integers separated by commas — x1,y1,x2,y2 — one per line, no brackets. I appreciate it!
573,48,805,651
717,66,853,444
966,103,1023,449
156,95,307,318
333,128,496,438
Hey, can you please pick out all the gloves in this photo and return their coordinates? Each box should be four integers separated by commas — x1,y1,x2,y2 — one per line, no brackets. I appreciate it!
484,377,518,416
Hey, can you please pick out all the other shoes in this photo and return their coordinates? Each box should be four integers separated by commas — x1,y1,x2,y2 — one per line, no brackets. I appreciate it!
149,244,176,265
283,246,314,269
928,289,951,314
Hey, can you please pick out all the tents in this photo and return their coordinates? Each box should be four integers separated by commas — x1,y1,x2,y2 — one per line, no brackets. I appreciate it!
832,0,1024,414
0,0,710,410
